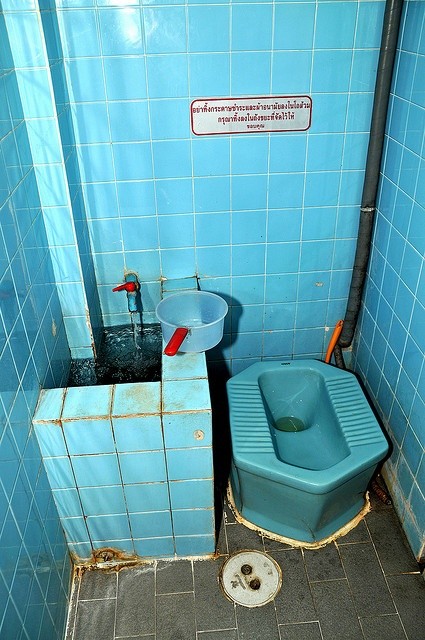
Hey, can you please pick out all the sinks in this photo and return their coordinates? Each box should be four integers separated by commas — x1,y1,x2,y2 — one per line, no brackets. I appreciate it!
31,274,219,567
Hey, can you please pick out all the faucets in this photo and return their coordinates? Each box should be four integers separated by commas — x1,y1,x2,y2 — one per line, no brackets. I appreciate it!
110,273,142,314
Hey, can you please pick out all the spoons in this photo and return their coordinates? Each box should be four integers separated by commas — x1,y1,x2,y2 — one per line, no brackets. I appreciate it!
155,291,228,356
